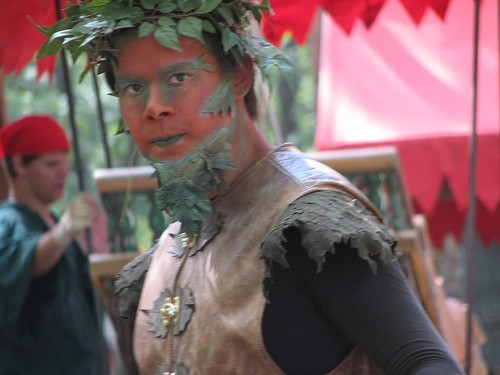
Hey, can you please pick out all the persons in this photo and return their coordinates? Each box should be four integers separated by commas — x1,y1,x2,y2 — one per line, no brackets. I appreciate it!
29,0,466,375
0,115,112,375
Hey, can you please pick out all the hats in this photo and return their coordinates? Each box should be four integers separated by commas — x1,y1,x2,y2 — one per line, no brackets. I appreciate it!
0,115,72,157
